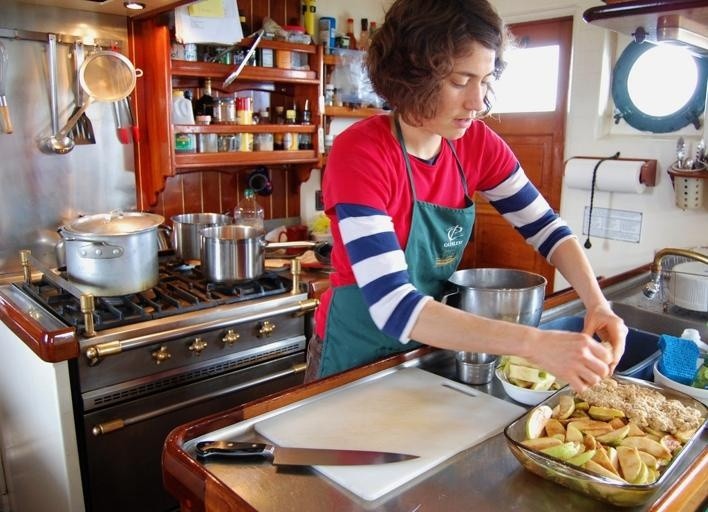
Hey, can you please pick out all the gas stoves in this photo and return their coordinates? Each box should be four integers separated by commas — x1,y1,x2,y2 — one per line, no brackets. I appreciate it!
9,256,309,394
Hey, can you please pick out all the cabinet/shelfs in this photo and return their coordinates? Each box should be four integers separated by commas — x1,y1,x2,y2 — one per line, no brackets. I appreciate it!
129,0,324,169
319,16,390,116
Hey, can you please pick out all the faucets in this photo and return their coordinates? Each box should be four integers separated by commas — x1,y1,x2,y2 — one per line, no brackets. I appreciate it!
641,248,708,299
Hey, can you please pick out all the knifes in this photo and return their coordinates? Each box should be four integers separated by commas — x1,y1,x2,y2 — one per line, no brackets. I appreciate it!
193,439,420,469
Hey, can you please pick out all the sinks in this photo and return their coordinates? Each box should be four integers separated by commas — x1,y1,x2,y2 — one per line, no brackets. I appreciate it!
575,301,708,345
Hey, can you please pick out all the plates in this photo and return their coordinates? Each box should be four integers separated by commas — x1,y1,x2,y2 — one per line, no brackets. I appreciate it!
492,365,560,406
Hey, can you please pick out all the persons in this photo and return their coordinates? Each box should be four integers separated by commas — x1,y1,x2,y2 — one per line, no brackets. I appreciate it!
300,1,633,394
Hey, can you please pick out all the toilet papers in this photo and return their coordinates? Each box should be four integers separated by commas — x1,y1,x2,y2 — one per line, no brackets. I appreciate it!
563,157,646,196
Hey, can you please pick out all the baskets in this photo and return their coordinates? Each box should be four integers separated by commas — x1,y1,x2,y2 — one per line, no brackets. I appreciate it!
667,159,708,211
649,247,708,320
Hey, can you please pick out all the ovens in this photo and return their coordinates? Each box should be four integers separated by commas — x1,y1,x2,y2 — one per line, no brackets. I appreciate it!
78,336,307,512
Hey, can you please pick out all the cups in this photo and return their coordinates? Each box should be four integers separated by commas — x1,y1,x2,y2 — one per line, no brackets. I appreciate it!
283,132,298,150
218,133,253,152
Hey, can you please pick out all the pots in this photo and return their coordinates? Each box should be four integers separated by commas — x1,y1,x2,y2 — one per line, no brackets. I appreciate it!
198,225,329,286
49,208,167,298
157,211,234,264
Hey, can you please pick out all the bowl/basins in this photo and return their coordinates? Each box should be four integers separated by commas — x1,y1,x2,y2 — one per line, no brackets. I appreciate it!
455,352,499,384
442,267,546,329
661,260,708,313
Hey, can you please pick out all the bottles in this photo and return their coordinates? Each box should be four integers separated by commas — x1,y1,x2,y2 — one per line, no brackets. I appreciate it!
195,75,311,125
233,189,264,228
324,83,343,108
345,16,376,49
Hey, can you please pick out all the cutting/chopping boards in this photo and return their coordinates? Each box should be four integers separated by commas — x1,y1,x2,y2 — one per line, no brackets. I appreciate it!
251,366,527,506
